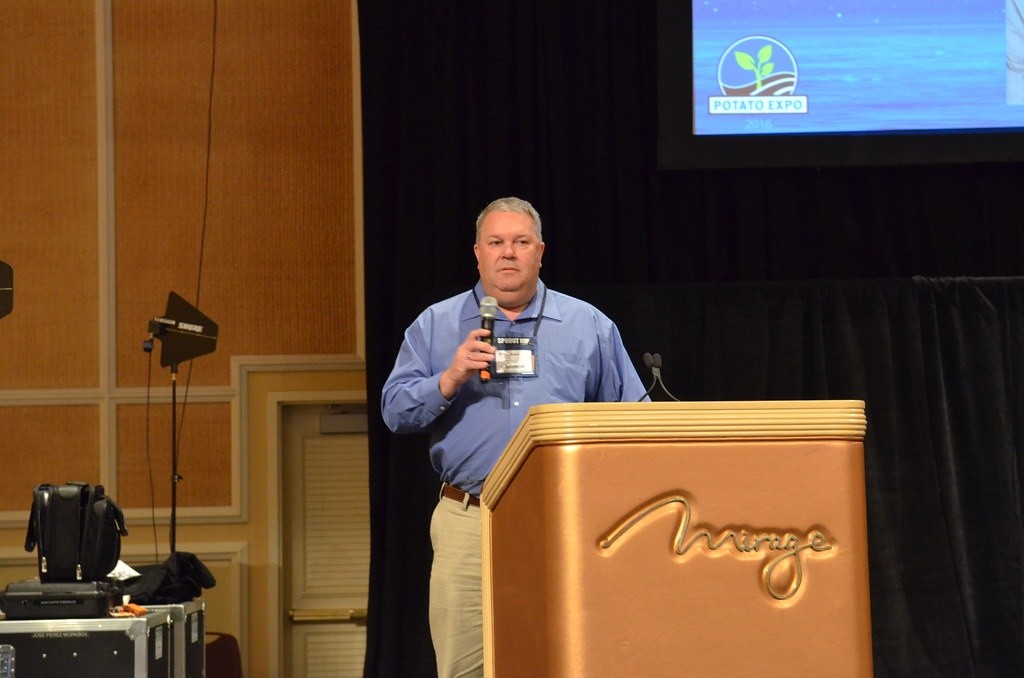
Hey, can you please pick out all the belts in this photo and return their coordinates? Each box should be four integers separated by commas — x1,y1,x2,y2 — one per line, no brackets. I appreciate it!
441,484,480,507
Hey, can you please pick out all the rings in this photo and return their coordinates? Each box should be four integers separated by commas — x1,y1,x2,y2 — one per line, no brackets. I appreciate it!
468,352,472,359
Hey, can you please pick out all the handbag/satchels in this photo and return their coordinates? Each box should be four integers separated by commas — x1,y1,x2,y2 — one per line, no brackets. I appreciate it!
24,481,127,582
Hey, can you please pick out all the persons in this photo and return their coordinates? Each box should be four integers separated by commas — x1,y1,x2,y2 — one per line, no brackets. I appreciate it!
380,198,652,678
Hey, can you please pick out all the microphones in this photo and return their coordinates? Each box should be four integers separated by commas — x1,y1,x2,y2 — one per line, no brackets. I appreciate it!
638,352,679,404
480,297,499,385
